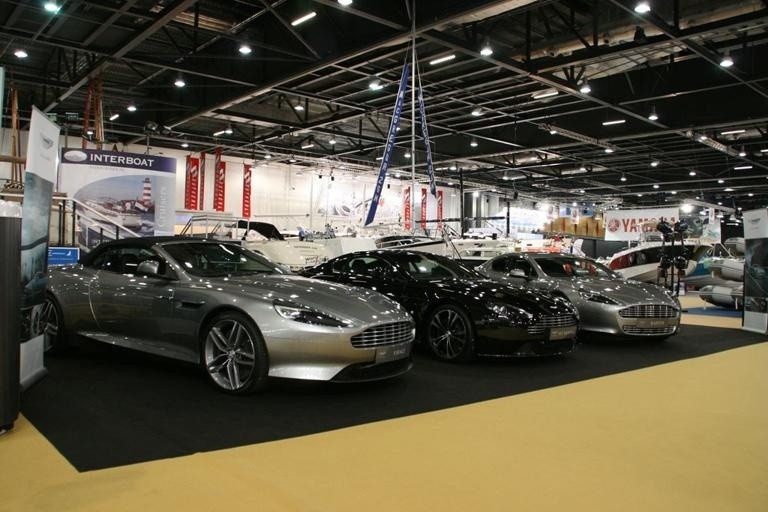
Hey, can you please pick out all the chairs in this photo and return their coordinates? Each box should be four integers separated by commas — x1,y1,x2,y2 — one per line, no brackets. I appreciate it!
541,261,565,274
353,260,368,275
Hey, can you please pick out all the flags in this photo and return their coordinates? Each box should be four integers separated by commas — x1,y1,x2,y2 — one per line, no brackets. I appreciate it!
134,197,150,214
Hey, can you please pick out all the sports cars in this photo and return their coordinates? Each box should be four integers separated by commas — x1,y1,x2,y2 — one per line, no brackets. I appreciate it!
213,219,331,274
19,237,416,398
299,249,582,361
473,251,681,340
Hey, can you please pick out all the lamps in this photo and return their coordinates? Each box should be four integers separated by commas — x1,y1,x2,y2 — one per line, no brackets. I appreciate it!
719,49,735,68
292,2,316,27
373,147,384,162
369,78,384,91
174,65,186,88
433,165,507,199
649,130,764,211
290,158,296,163
472,104,483,117
265,154,273,160
404,148,411,160
42,2,61,15
516,156,600,209
480,31,494,57
239,25,252,55
108,99,137,122
86,129,94,138
339,1,355,7
549,126,558,134
530,88,559,100
428,54,456,65
469,136,478,147
213,117,233,136
620,171,628,182
603,144,615,154
14,49,29,59
634,2,651,13
301,135,314,151
294,97,305,110
580,77,592,94
328,126,337,146
181,134,191,150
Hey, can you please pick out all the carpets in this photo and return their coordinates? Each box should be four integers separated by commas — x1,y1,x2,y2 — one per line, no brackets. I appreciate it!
21,323,768,473
